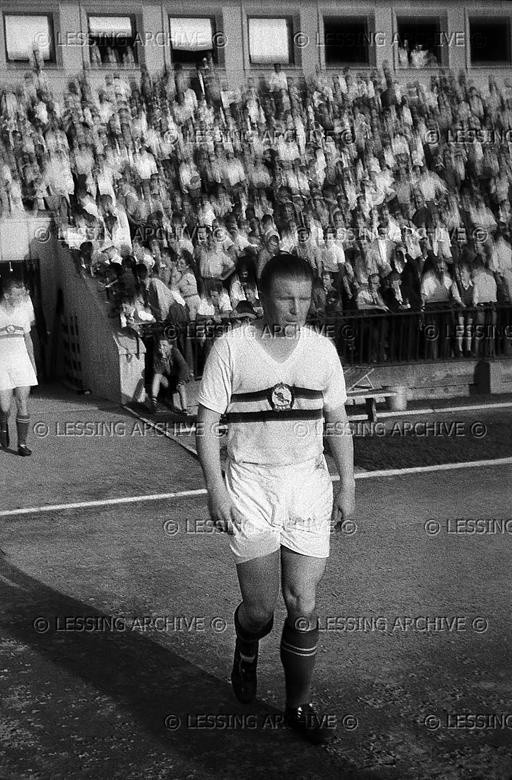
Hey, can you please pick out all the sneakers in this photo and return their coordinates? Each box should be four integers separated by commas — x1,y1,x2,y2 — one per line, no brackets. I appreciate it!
230,636,260,705
0,421,10,448
17,443,32,456
282,702,336,745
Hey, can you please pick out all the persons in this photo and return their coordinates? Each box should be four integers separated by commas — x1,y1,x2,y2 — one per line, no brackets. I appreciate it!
195,253,359,744
0,277,39,456
0,33,511,418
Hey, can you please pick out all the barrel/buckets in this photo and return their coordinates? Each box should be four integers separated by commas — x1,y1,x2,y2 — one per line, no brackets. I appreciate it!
382,384,409,411
